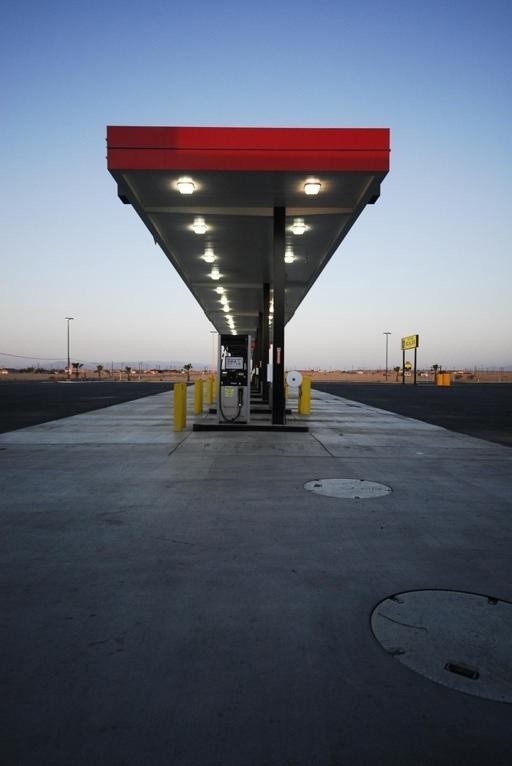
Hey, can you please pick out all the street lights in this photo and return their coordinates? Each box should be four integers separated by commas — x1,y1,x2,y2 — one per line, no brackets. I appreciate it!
382,332,391,381
65,316,76,369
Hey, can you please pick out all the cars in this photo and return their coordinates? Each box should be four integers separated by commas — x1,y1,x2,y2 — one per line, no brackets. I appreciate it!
399,369,464,378
125,369,161,375
